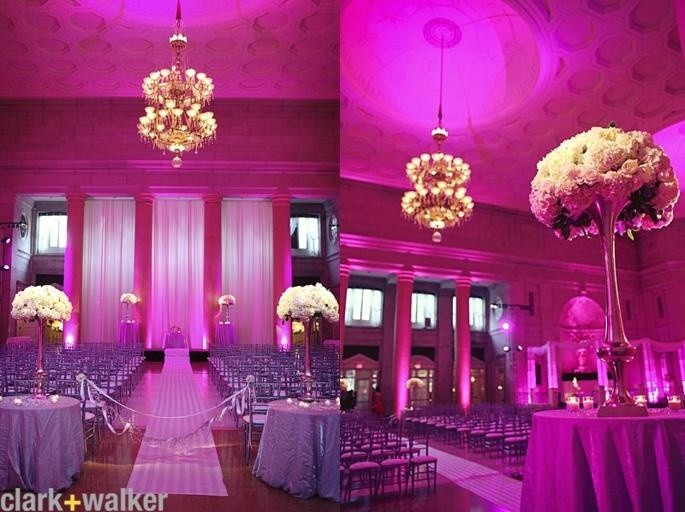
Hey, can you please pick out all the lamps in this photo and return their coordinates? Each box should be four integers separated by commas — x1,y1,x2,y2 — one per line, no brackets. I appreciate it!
401,35,475,241
136,1,218,168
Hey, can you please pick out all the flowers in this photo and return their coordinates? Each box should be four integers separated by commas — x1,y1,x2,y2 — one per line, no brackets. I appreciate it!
276,283,339,324
529,120,680,242
120,292,141,305
10,285,73,332
218,293,236,306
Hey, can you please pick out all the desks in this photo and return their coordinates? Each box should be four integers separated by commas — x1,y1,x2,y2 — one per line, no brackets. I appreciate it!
0,394,84,495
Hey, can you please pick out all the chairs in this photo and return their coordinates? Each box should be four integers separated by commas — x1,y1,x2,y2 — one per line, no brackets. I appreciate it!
405,410,531,463
0,339,146,460
339,412,437,507
208,342,340,465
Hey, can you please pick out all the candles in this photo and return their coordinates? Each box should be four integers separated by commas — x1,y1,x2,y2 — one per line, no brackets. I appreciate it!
567,396,681,411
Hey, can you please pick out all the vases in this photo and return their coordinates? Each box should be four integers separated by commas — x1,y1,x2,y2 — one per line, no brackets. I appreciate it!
596,236,649,416
226,305,230,321
126,305,129,321
